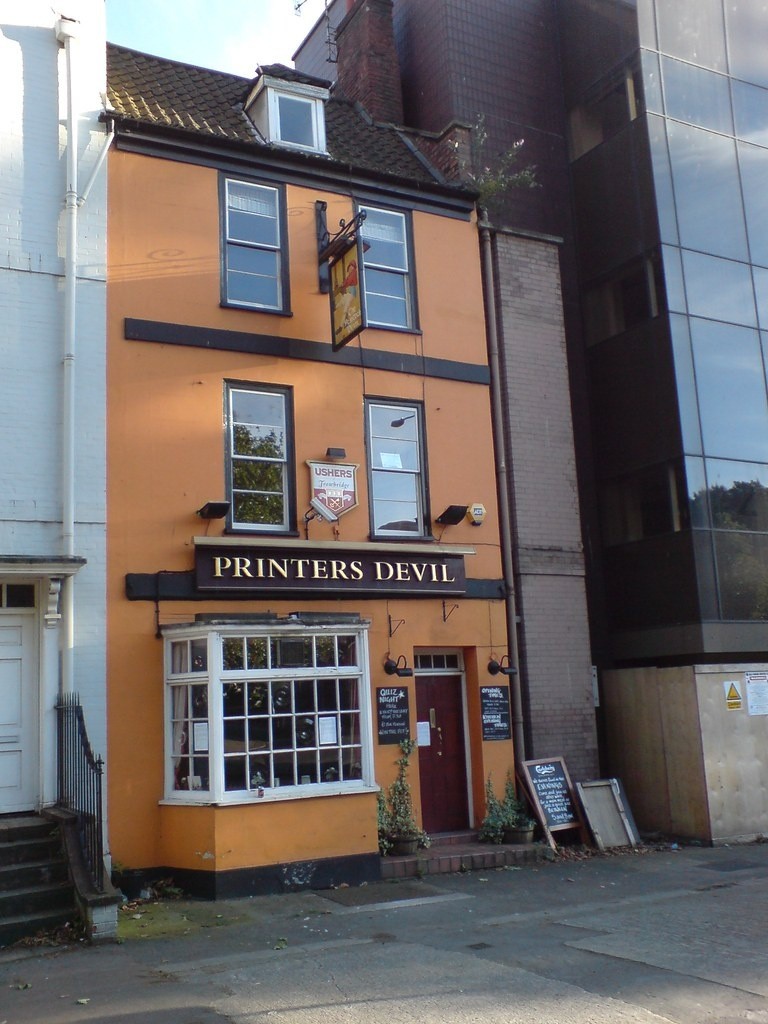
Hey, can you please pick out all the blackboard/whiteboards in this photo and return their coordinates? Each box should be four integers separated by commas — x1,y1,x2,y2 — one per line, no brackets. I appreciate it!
479,684,512,741
377,687,411,746
519,755,582,832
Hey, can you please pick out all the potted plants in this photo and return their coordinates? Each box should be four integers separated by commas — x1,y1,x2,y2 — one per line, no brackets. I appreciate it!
374,737,432,858
476,767,538,846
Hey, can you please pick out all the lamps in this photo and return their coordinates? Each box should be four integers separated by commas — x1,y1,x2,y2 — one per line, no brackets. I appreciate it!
195,501,231,519
486,654,519,676
436,504,470,528
384,654,414,677
324,448,348,459
